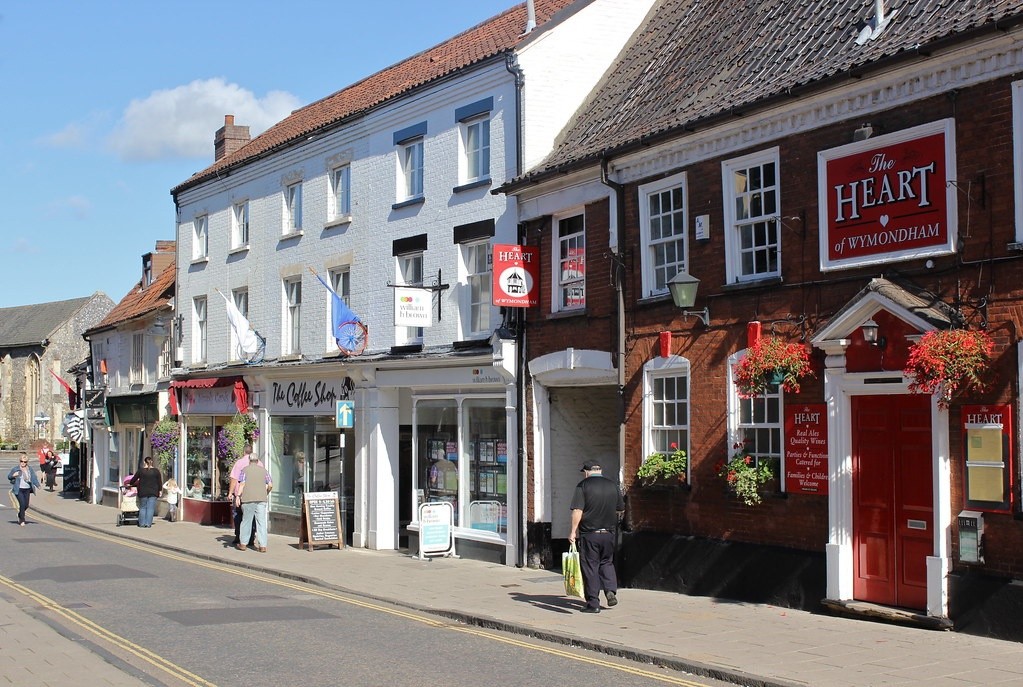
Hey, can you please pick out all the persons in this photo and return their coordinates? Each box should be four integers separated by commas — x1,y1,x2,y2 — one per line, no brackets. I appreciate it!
430,449,458,498
227,445,264,547
292,452,313,493
126,456,163,527
567,460,626,614
69,441,79,466
187,478,206,499
235,453,273,552
38,444,58,492
163,478,183,522
8,455,41,526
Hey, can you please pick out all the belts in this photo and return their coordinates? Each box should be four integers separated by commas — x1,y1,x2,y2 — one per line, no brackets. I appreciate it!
581,530,612,534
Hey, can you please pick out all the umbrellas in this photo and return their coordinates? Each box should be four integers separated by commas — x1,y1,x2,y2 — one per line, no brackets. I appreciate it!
29,438,54,459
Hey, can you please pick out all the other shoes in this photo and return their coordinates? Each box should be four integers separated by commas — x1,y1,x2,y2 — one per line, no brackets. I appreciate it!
16,513,22,523
246,542,254,548
580,603,600,613
259,547,267,552
20,521,25,526
231,536,240,545
606,591,618,607
140,524,152,528
237,543,246,550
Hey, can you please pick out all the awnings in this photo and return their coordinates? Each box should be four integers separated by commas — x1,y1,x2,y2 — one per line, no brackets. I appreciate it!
61,409,84,444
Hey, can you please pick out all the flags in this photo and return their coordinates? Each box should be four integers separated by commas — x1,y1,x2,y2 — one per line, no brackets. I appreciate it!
218,290,257,355
49,368,78,411
316,274,361,352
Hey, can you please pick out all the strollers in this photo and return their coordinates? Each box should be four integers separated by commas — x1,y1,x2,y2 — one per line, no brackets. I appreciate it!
116,474,139,526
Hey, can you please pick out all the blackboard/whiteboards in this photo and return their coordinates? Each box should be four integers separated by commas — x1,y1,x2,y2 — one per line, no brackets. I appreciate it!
300,492,343,544
63,465,82,492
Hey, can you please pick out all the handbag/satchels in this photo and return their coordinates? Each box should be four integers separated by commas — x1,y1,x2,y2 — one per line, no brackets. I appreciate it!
562,540,584,599
10,478,16,484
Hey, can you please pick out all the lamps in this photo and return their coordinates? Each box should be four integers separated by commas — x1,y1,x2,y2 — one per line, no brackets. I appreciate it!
860,317,887,349
852,122,881,141
666,266,710,326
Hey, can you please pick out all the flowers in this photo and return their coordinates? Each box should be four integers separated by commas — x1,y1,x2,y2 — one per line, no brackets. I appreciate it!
905,328,993,403
219,419,259,478
638,442,686,478
149,416,181,468
716,435,773,502
730,339,812,394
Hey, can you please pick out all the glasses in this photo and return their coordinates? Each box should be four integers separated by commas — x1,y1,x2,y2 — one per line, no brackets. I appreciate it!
20,461,27,463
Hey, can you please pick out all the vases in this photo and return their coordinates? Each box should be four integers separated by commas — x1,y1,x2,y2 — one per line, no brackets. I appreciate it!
641,475,681,485
765,367,785,385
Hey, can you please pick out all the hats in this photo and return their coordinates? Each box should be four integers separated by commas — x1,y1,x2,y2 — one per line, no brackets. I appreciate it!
579,459,602,472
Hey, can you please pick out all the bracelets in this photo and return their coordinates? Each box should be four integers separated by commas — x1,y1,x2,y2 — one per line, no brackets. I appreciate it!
237,495,240,498
